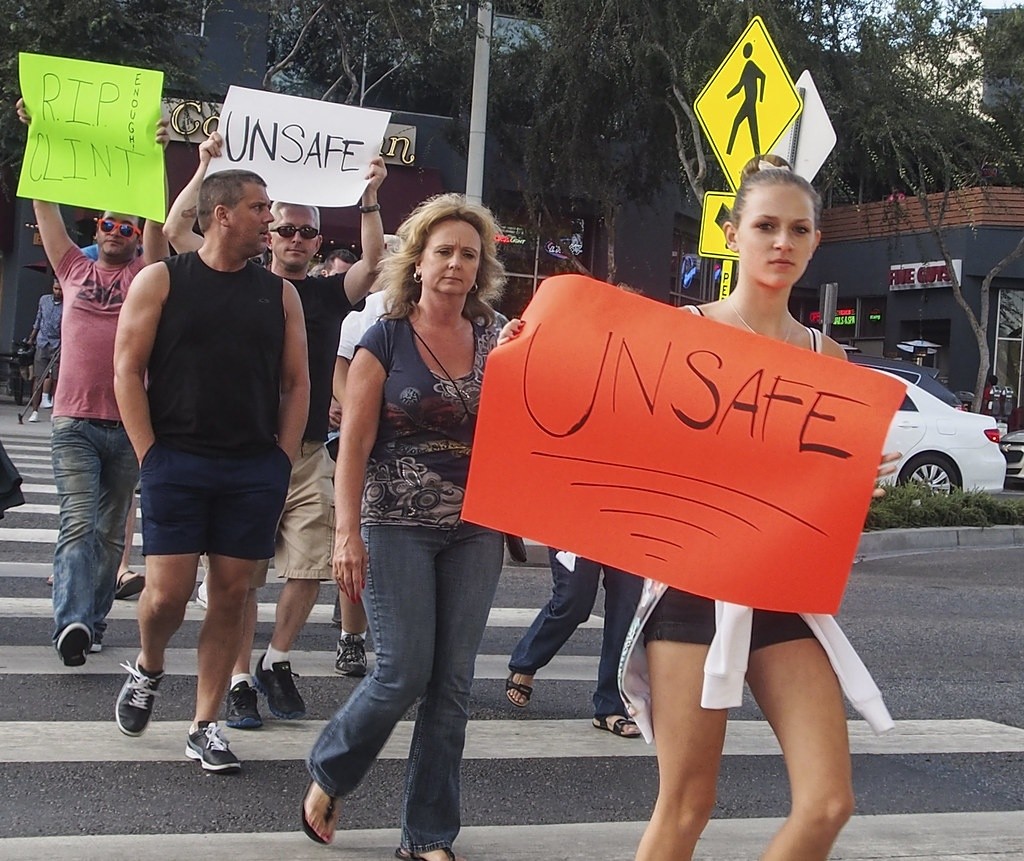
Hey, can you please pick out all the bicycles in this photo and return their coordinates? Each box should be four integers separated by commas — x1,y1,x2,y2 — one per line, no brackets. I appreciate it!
1,352,24,405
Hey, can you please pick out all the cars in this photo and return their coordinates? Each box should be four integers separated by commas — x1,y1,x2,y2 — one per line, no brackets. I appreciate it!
857,368,1007,498
998,429,1024,482
847,353,968,411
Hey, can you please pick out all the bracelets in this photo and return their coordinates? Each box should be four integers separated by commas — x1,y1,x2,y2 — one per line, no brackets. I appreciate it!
358,202,381,213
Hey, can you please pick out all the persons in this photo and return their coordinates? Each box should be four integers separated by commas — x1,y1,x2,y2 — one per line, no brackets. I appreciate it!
161,131,390,729
981,374,1002,416
17,96,173,669
496,167,905,861
504,284,646,739
297,192,509,860
111,168,311,771
309,229,401,682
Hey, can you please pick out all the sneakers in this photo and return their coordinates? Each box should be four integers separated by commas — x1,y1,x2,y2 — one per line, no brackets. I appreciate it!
334,631,368,675
252,652,306,719
115,652,165,736
226,680,262,728
90,642,102,653
57,622,92,667
330,595,342,623
185,720,241,772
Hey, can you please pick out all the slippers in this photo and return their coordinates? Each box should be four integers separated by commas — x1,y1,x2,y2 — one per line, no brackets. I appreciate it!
395,841,455,861
300,776,335,845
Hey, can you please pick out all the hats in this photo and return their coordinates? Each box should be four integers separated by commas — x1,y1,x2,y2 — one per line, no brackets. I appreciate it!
383,234,403,256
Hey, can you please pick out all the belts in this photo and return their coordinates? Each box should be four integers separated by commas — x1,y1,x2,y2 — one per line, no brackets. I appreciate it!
74,417,123,428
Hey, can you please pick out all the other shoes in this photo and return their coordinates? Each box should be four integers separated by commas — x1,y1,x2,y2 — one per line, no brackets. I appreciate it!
40,401,53,408
28,411,39,422
506,533,528,562
197,580,208,609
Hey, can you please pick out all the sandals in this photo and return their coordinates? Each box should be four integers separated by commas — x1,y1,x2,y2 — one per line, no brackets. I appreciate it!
592,713,641,738
505,670,534,708
114,570,145,599
47,575,54,584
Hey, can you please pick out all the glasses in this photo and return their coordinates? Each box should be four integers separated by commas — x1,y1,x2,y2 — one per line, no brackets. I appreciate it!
270,225,320,239
98,219,142,239
